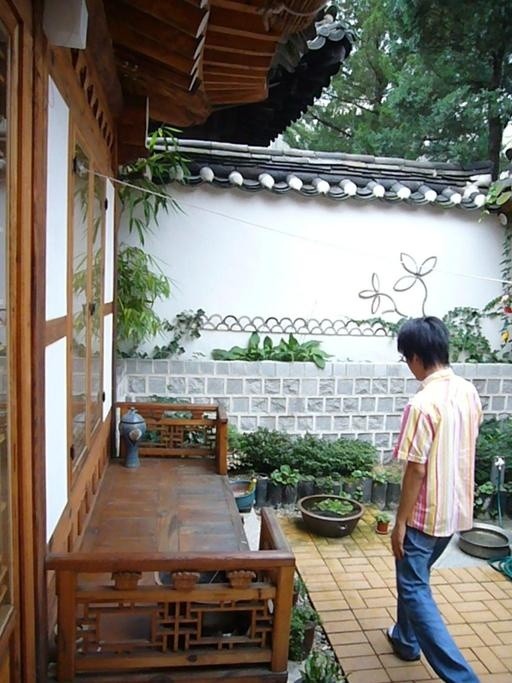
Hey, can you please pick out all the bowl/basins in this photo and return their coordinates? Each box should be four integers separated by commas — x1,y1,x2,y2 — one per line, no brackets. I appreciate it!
229,478,258,509
457,522,511,560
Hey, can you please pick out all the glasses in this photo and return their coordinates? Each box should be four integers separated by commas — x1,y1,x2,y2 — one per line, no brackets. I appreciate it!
401,348,409,363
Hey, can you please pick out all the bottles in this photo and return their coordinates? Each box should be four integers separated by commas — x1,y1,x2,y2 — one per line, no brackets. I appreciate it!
113,404,153,470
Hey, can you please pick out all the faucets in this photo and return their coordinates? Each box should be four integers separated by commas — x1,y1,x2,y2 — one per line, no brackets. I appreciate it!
495,458,506,470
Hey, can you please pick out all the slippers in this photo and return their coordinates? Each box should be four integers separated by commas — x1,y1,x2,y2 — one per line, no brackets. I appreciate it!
386,623,421,661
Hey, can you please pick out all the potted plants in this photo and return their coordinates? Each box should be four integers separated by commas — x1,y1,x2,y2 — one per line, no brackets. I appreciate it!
371,510,393,534
269,576,308,607
288,601,324,661
292,648,353,683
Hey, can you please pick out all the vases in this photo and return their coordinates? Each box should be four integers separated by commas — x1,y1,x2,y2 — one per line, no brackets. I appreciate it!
118,407,147,468
230,479,256,513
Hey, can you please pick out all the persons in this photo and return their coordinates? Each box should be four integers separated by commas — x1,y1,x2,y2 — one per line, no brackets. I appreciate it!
383,314,484,682
